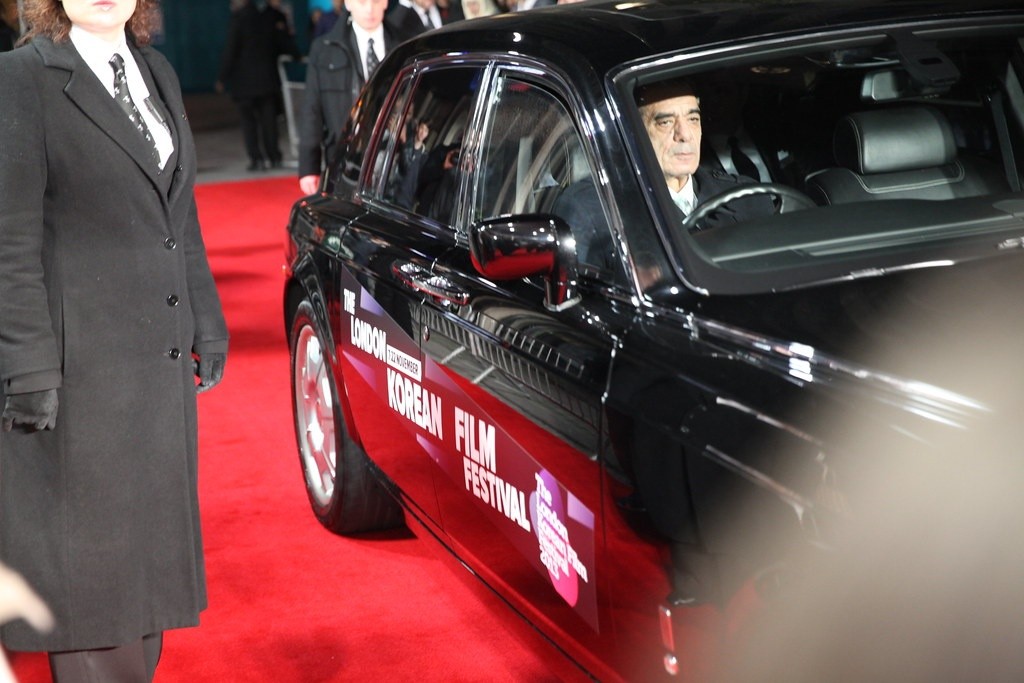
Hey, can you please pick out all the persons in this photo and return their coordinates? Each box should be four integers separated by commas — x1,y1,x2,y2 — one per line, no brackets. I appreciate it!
544,85,781,283
393,117,465,225
297,0,557,197
0,0,231,683
214,0,308,170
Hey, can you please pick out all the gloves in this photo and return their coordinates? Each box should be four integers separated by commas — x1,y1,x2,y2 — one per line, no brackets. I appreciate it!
2,386,59,433
192,353,225,394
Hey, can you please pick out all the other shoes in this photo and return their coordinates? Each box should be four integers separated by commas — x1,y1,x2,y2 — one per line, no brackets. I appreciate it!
248,159,266,172
273,156,281,171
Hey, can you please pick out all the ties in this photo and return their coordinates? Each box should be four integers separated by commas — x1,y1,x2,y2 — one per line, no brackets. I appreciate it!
368,38,381,79
425,9,436,30
109,54,160,169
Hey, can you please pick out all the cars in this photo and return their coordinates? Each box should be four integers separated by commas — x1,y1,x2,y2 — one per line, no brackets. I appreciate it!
280,0,1024,682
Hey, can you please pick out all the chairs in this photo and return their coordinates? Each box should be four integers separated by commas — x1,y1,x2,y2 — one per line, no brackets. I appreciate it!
806,102,1006,206
